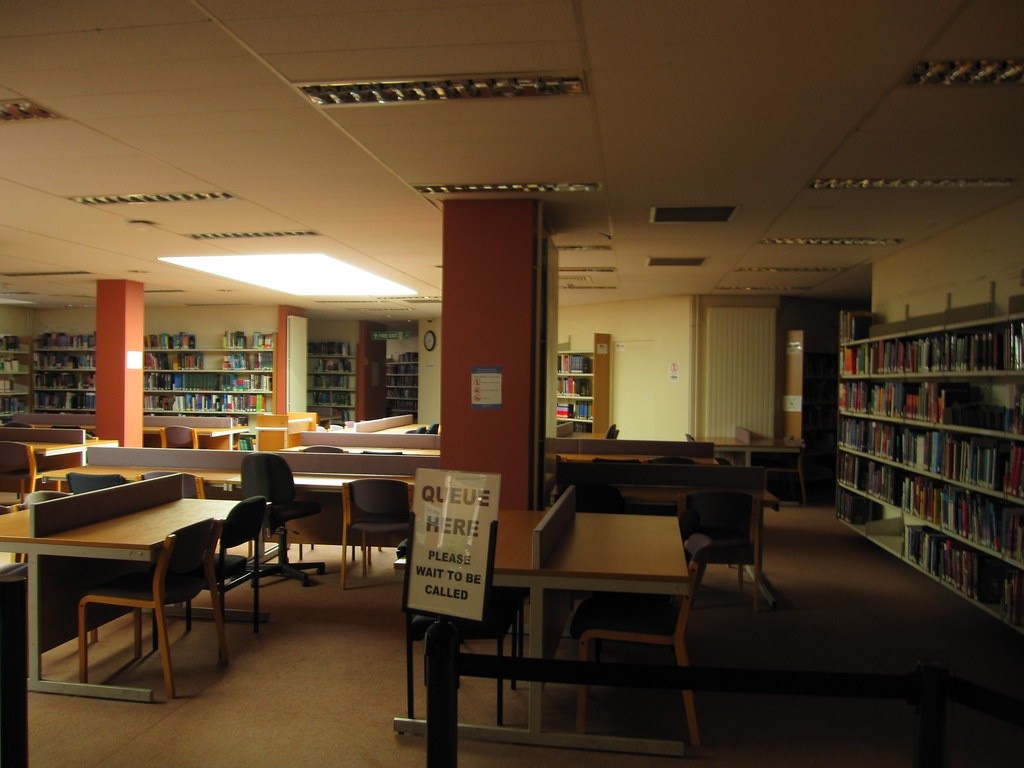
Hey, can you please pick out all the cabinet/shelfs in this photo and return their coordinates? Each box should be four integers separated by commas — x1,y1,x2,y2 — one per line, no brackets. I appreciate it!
0,350,33,414
305,355,356,428
554,332,613,430
384,360,418,421
803,350,838,481
834,313,1023,633
32,347,96,413
142,348,275,416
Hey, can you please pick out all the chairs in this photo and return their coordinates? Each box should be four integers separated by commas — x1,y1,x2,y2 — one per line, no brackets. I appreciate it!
0,423,702,760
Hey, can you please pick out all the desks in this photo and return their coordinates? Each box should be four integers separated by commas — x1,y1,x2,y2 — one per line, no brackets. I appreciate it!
1,412,807,755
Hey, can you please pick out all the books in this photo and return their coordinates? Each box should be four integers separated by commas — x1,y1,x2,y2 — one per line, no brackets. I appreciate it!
144,331,274,449
836,311,1024,632
557,355,593,433
308,340,351,426
0,332,95,423
385,352,418,420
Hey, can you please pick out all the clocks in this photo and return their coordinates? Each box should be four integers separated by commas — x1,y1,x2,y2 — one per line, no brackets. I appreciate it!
423,330,435,350
